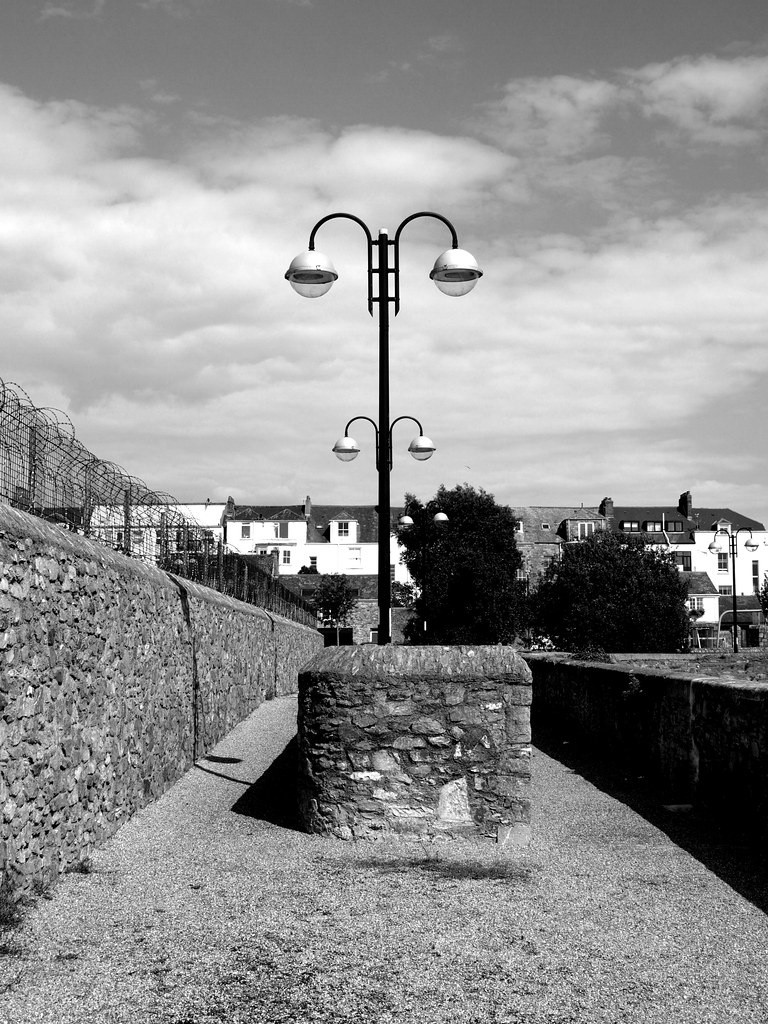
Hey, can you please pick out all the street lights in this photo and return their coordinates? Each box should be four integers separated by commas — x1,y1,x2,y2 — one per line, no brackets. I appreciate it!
707,526,759,651
396,500,451,643
282,212,483,637
